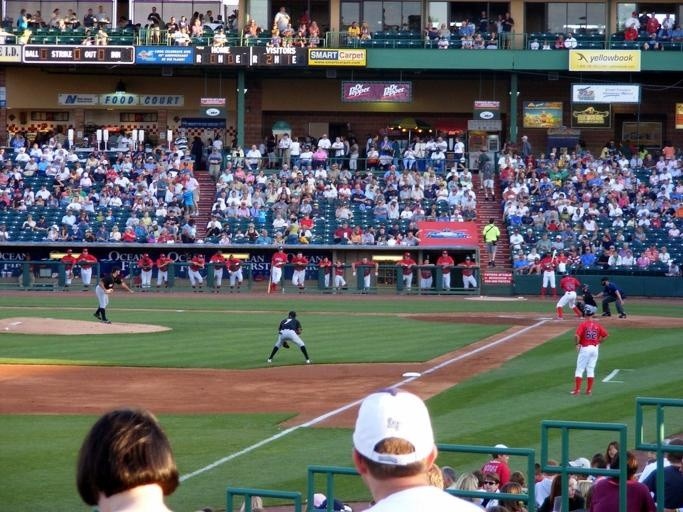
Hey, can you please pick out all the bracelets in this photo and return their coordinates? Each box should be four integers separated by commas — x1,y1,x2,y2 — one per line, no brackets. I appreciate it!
575,343,579,346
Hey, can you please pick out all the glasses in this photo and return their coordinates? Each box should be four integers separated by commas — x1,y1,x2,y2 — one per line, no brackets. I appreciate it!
484,481,495,485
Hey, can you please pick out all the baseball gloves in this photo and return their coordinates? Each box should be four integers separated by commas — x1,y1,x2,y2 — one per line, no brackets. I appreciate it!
105,288,112,295
283,342,288,349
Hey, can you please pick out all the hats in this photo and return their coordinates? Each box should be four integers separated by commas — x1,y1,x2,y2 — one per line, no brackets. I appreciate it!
568,457,591,469
352,387,435,465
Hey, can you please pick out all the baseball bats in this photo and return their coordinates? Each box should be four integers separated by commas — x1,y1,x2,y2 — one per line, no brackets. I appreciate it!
550,249,557,262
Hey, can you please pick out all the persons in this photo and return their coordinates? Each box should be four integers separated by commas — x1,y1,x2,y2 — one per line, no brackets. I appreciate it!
94,268,133,324
625,9,683,50
75,389,683,512
1,6,372,46
0,124,477,289
477,136,683,277
424,10,576,50
268,311,311,364
570,305,609,396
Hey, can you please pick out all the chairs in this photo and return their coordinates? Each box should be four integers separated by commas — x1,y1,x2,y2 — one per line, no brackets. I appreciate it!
205,155,476,245
505,165,682,275
2,22,682,52
0,148,185,242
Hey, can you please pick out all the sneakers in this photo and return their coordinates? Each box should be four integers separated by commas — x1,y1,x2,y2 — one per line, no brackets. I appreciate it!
620,314,626,318
602,313,611,315
